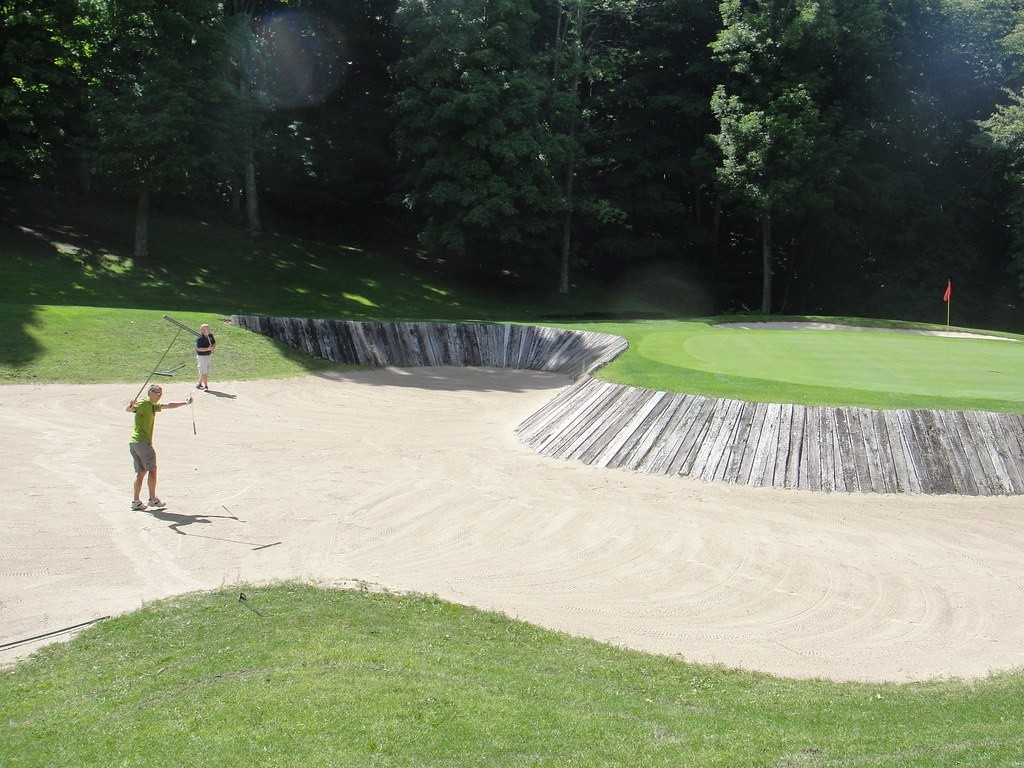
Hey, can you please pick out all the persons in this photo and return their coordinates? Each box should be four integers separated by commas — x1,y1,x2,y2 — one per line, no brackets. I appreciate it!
126,384,193,511
195,324,217,392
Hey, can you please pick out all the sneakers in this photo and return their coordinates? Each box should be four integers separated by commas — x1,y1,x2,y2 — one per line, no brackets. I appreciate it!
147,496,166,507
130,500,148,510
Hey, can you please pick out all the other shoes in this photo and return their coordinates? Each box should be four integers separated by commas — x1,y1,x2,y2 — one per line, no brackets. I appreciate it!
203,386,208,392
196,384,202,388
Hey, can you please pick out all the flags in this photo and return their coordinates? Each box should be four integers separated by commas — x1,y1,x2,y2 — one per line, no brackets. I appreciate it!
944,281,951,301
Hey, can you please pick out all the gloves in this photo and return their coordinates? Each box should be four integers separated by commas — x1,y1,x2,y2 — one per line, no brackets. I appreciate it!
186,397,193,405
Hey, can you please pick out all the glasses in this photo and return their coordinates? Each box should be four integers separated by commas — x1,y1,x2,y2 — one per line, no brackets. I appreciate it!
152,390,162,398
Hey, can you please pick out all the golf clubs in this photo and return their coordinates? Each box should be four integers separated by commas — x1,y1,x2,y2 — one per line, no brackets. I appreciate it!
204,327,215,353
187,397,196,435
